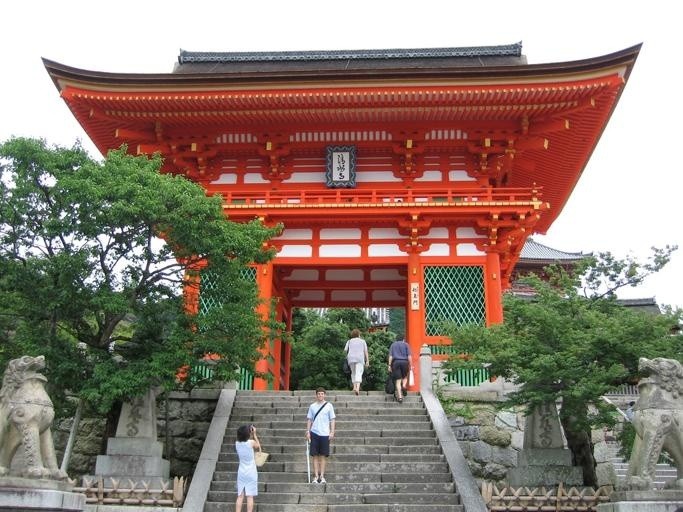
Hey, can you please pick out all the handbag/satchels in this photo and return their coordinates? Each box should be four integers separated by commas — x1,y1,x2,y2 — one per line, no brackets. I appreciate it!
385,371,396,395
254,437,270,468
342,358,352,377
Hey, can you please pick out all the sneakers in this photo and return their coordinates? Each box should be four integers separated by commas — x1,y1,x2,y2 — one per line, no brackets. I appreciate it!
352,389,359,396
311,475,327,484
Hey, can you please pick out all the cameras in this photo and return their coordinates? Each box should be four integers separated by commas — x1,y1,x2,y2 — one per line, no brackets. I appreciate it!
249,424,254,431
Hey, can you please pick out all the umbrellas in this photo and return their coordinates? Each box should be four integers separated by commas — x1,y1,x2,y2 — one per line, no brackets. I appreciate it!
305,430,311,483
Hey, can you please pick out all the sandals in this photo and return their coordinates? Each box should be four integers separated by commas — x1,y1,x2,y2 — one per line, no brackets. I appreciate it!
396,386,408,402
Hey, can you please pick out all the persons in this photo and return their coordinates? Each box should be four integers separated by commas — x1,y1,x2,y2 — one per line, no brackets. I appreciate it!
384,334,415,402
233,423,260,511
342,329,369,394
305,386,336,483
626,400,635,421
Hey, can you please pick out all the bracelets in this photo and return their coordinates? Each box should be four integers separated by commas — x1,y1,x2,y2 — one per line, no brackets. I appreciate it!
330,431,335,435
388,364,391,366
